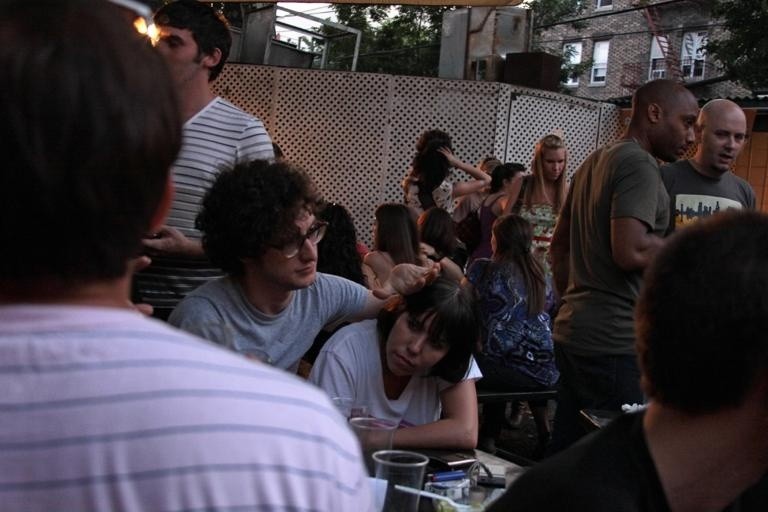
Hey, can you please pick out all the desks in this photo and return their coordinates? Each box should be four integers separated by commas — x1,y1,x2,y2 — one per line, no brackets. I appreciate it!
369,445,527,512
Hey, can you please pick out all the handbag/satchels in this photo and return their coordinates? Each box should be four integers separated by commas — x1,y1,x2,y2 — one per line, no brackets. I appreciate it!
458,208,483,254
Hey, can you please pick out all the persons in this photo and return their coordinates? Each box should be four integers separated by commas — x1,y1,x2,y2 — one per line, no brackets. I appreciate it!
174,158,436,375
304,281,487,455
542,77,701,444
129,1,274,331
0,1,378,511
301,116,579,461
487,207,768,512
662,97,757,231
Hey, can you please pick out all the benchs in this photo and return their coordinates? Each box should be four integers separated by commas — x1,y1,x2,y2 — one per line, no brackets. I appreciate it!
477,387,557,403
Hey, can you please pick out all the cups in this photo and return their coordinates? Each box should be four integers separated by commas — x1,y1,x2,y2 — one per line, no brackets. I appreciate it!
370,447,431,512
348,415,399,478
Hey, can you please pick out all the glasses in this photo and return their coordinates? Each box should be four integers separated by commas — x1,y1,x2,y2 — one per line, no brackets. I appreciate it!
276,220,331,259
